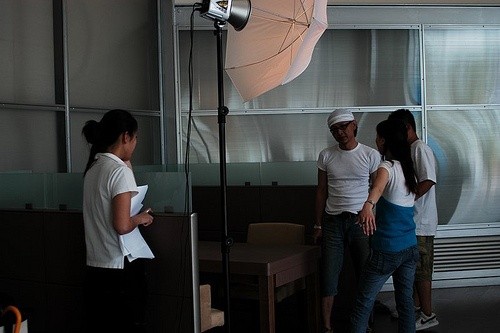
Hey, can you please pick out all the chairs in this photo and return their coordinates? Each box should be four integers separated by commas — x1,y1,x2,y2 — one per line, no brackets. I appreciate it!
232,223,307,301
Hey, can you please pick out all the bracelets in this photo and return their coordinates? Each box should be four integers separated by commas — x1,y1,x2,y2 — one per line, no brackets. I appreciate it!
313,225,322,230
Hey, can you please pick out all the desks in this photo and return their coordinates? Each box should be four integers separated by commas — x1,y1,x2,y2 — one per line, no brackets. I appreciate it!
196,240,322,333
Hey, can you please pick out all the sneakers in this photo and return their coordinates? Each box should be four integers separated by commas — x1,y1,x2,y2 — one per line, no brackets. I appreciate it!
413,311,439,330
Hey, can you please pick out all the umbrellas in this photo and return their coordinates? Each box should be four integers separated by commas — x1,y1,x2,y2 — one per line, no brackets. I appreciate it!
222,0,329,104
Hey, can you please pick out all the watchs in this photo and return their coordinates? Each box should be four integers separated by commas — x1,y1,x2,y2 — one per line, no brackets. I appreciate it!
365,200,376,209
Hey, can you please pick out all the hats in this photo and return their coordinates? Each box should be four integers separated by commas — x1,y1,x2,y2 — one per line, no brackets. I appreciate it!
328,109,354,128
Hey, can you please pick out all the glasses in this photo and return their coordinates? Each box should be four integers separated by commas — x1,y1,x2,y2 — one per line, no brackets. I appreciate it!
330,120,355,132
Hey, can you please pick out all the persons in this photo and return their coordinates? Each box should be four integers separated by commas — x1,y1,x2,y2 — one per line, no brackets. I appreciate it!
83,143,133,179
310,109,382,333
348,119,417,332
387,108,440,331
78,108,159,333
